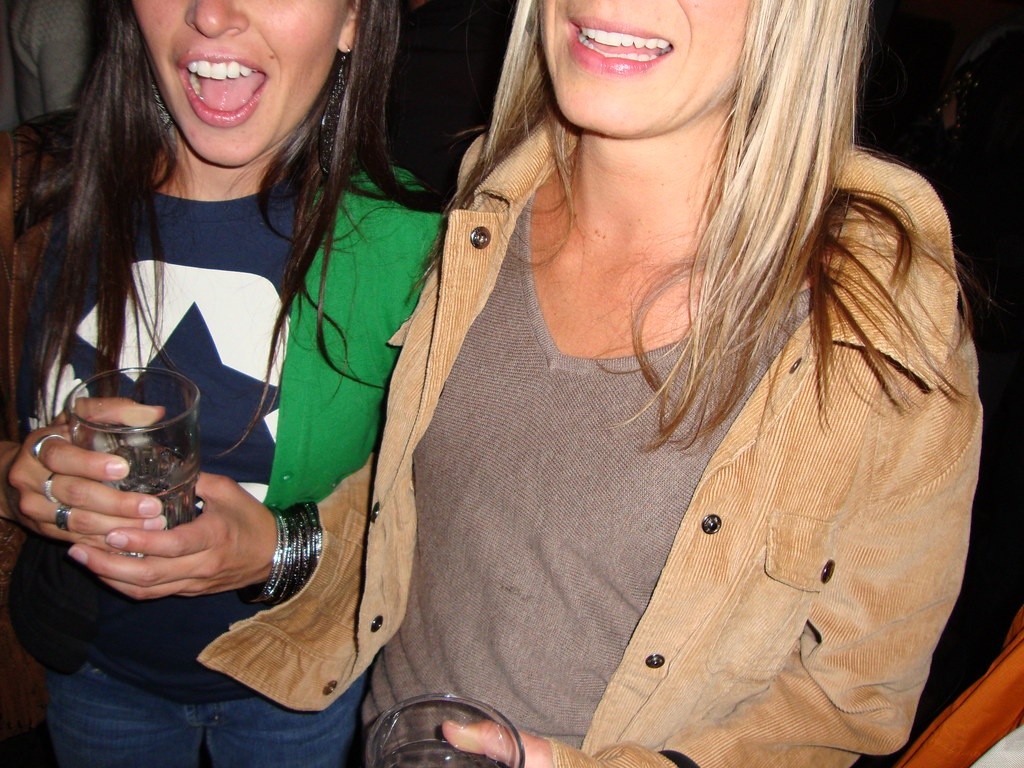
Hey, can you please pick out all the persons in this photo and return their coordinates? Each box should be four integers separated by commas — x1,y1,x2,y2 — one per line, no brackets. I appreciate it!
0,0,443,768
197,0,982,768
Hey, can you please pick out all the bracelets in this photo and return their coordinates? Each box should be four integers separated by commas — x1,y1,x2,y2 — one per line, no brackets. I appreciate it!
233,501,323,606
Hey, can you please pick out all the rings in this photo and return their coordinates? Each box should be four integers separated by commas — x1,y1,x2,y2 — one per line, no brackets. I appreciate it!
32,434,65,458
55,503,72,531
45,472,58,503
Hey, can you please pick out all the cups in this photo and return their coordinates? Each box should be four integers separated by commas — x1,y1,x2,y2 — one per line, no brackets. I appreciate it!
63,365,201,529
365,694,526,768
